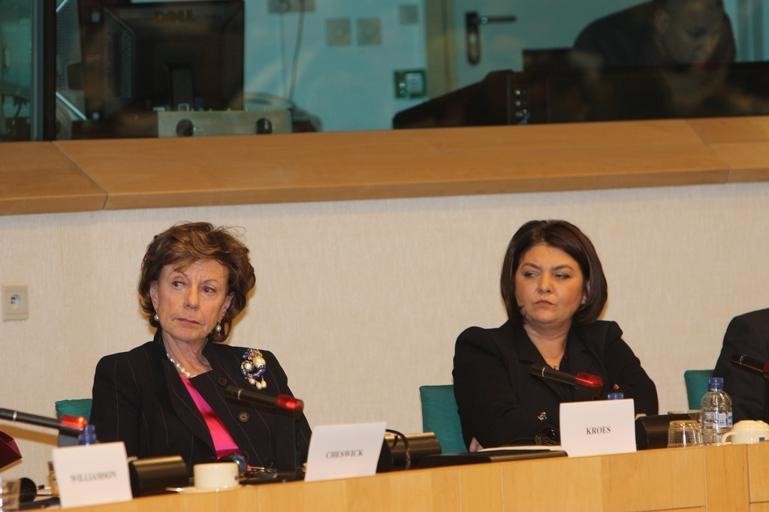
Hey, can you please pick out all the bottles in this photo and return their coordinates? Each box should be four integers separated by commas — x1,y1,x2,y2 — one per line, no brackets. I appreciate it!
78,425,97,446
701,375,734,445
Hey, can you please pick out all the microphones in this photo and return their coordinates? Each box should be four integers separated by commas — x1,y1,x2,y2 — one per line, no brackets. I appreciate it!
1,407,87,438
728,354,769,379
226,384,304,415
529,362,605,396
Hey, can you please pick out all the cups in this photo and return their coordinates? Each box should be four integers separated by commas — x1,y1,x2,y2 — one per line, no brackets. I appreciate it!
666,419,701,449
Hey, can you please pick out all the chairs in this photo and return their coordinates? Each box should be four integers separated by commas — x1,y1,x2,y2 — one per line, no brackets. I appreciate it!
684,369,714,409
420,385,468,454
54,399,93,423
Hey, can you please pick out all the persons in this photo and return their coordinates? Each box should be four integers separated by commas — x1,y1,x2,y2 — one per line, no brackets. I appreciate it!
89,222,311,473
714,308,769,426
452,219,659,452
574,0,735,120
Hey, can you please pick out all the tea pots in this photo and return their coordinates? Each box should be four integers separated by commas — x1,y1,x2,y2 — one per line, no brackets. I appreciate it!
721,419,769,444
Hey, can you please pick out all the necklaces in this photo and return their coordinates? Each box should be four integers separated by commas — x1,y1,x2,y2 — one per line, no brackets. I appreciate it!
167,351,194,379
554,364,559,370
